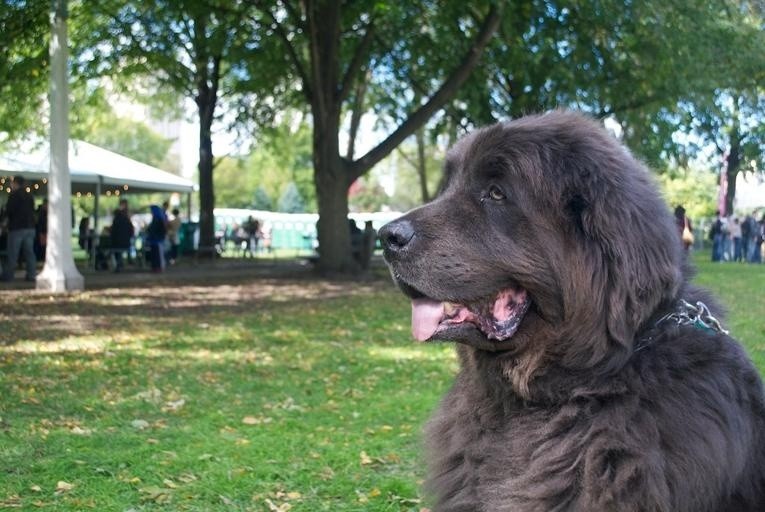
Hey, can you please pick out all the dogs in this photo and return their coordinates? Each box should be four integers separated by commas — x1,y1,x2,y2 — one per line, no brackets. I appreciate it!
377,104,765,512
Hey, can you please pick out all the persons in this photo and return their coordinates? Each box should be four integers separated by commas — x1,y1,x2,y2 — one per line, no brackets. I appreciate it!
675,206,765,263
